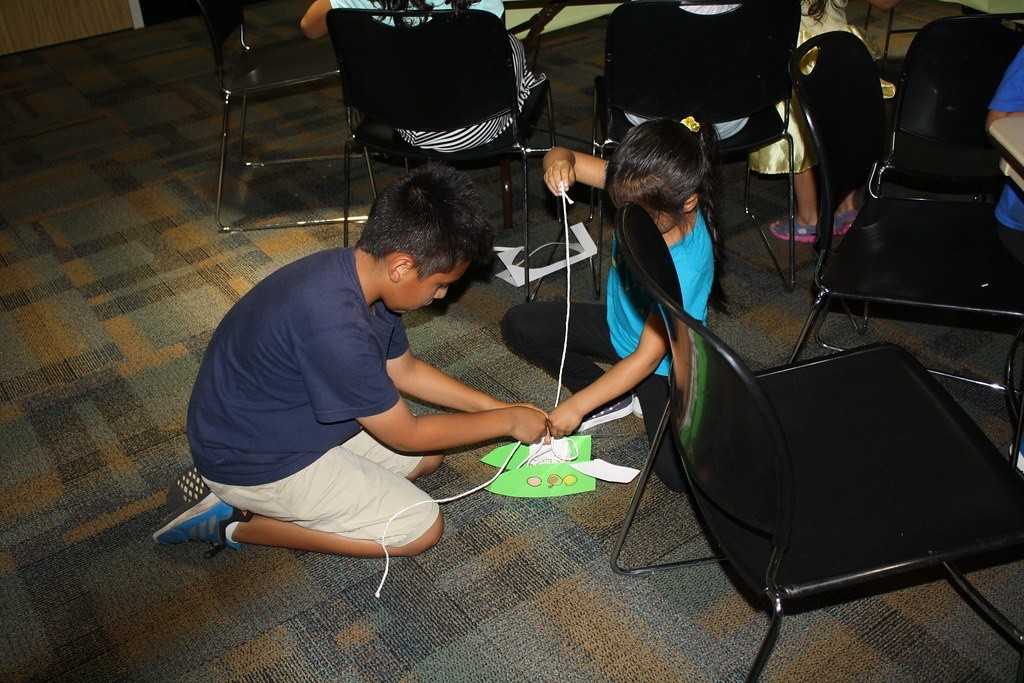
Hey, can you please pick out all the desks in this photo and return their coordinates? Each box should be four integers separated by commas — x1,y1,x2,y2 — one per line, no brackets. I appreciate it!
494,0,631,227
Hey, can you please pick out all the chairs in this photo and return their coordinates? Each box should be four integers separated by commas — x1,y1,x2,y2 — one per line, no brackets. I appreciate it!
321,7,568,306
593,0,805,301
782,29,1024,472
611,198,1024,683
885,13,1024,194
189,0,383,232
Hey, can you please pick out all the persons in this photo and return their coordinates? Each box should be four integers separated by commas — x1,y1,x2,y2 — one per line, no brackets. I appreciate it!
984,41,1024,265
624,0,749,141
153,164,551,561
498,116,716,439
745,0,896,243
300,0,543,159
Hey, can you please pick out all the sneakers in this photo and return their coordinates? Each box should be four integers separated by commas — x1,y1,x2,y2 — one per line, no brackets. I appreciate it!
769,212,819,244
151,463,253,561
832,209,859,234
574,389,636,432
